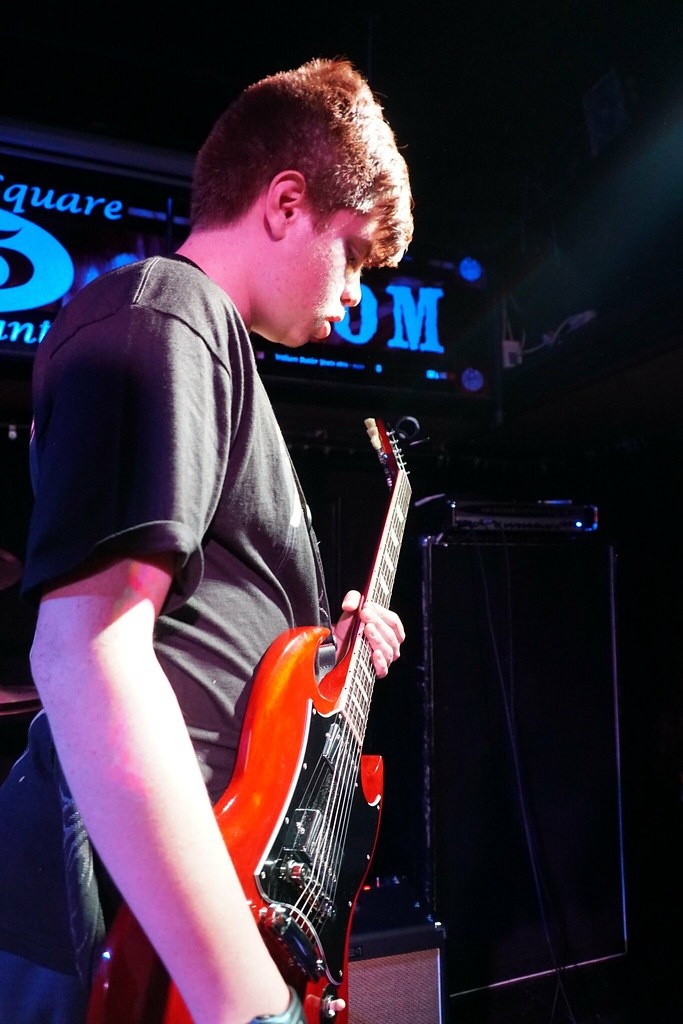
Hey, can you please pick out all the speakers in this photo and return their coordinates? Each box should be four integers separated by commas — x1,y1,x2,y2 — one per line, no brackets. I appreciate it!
320,911,443,1024
386,525,627,1001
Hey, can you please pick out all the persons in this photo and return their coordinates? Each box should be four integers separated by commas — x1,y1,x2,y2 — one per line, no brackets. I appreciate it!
0,57,414,1024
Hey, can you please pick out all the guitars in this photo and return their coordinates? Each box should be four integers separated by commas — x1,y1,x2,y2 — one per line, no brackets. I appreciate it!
81,408,431,1024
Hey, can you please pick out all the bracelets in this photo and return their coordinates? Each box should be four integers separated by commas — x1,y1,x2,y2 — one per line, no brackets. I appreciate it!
247,985,306,1024
